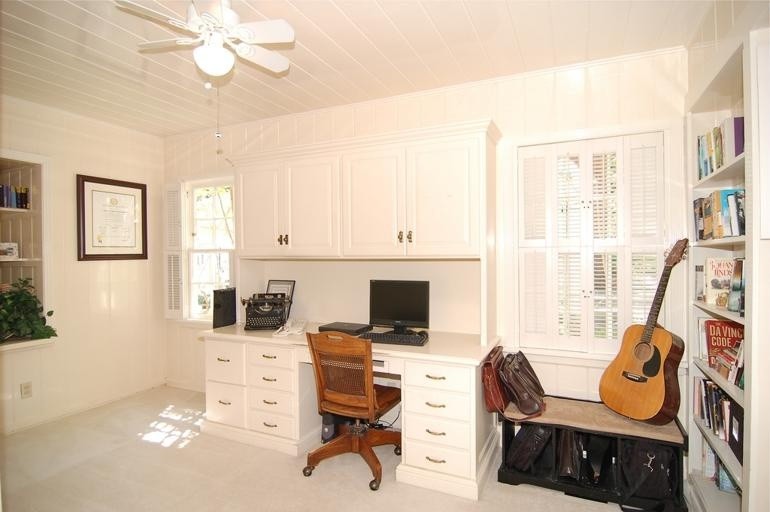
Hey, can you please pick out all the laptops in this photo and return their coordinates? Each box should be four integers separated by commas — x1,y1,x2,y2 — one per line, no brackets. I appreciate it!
318,321,373,336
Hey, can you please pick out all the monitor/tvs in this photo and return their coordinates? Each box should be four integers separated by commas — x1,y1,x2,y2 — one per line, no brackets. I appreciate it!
369,280,430,335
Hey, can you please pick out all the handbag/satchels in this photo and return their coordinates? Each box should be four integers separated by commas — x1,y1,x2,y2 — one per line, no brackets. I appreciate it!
482,345,514,414
557,429,678,501
506,423,552,472
496,350,544,415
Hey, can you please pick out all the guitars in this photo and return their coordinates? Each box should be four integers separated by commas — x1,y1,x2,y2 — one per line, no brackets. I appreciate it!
600,239,689,424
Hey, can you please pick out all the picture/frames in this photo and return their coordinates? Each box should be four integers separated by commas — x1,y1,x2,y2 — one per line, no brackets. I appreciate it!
76,174,146,262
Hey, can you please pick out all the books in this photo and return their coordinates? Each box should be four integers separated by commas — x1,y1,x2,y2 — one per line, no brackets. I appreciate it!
0,184,31,209
692,116,746,497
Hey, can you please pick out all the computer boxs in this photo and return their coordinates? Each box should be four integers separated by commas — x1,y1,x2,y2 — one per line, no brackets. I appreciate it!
321,414,356,445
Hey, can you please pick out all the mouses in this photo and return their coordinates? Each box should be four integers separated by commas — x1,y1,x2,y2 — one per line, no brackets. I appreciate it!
417,330,428,338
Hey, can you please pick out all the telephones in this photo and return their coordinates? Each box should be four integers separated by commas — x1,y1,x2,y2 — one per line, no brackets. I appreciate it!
284,318,306,331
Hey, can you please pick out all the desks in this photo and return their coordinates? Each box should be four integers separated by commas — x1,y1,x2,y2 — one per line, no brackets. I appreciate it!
497,395,689,510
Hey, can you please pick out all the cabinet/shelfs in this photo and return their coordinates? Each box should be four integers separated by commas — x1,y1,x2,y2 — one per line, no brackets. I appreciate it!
202,319,500,500
0,150,57,351
225,123,500,260
678,0,770,512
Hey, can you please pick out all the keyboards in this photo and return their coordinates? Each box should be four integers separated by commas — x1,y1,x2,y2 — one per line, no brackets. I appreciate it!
357,332,429,346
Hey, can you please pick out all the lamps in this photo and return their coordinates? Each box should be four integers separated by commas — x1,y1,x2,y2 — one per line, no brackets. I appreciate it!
192,43,235,79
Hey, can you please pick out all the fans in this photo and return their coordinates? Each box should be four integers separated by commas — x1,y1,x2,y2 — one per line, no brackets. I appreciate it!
113,1,297,74
